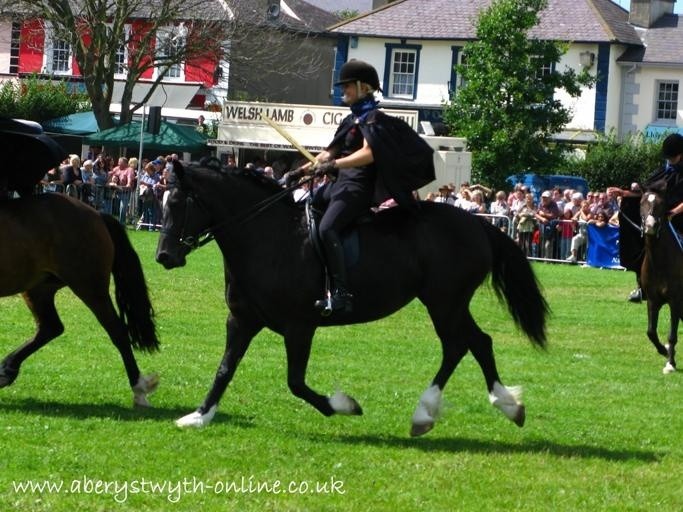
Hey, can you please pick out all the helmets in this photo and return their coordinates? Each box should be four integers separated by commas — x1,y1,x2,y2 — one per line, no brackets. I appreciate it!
333,61,384,93
659,133,683,159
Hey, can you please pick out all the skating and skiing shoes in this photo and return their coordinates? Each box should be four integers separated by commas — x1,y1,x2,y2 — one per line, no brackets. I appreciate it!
566,255,577,263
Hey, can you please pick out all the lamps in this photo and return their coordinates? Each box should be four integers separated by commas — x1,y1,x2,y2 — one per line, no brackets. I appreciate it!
580,51,595,66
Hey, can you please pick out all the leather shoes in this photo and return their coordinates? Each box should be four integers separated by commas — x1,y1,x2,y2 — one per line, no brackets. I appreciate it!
628,290,646,303
314,286,362,313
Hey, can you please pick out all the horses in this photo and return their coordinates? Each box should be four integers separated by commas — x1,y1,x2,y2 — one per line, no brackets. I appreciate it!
154,156,552,439
0,191,163,408
634,181,683,376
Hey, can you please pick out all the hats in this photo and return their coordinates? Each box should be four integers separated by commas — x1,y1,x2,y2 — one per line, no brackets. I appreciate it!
541,190,552,198
438,185,453,192
151,159,161,164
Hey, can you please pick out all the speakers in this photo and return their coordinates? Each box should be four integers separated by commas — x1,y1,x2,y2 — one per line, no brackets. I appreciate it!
146,106,161,135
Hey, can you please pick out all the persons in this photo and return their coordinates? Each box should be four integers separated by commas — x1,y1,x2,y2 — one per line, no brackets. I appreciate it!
629,132,682,305
282,60,392,319
26,147,651,273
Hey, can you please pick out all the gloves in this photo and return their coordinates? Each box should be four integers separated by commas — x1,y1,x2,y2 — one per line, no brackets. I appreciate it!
307,160,339,178
283,167,304,187
